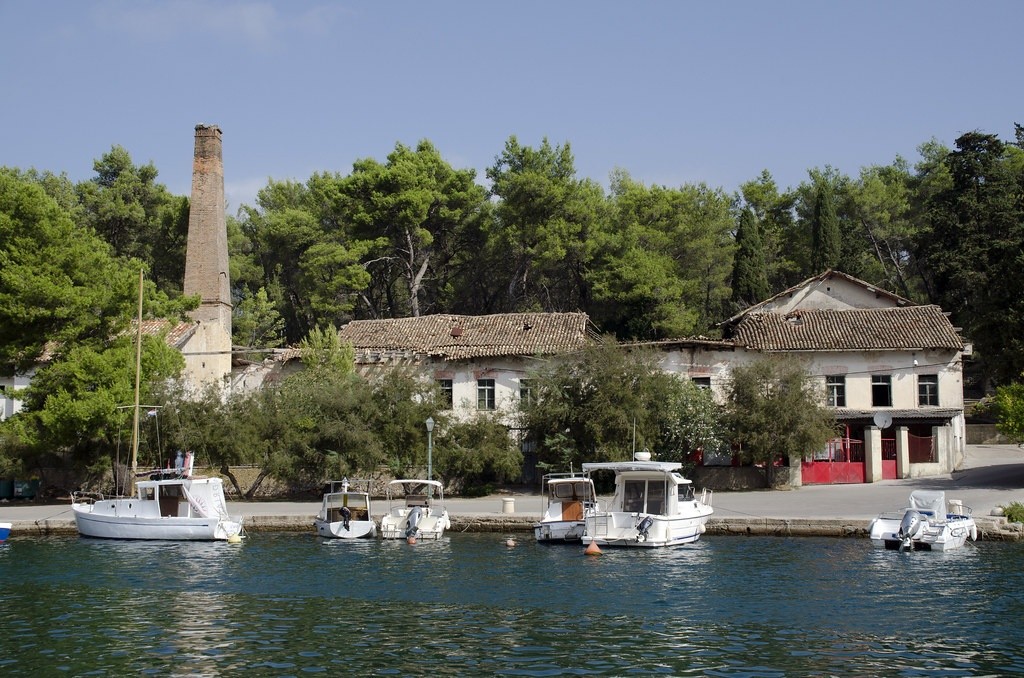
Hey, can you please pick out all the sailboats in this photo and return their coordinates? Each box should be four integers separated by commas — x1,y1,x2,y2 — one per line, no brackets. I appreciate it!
68,269,246,540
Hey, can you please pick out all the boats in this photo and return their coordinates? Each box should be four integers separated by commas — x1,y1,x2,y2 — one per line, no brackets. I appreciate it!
315,478,378,540
381,477,449,542
533,464,598,541
582,451,716,548
869,487,978,552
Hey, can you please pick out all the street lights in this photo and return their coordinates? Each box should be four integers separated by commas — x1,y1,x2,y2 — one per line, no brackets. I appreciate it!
425,415,436,503
